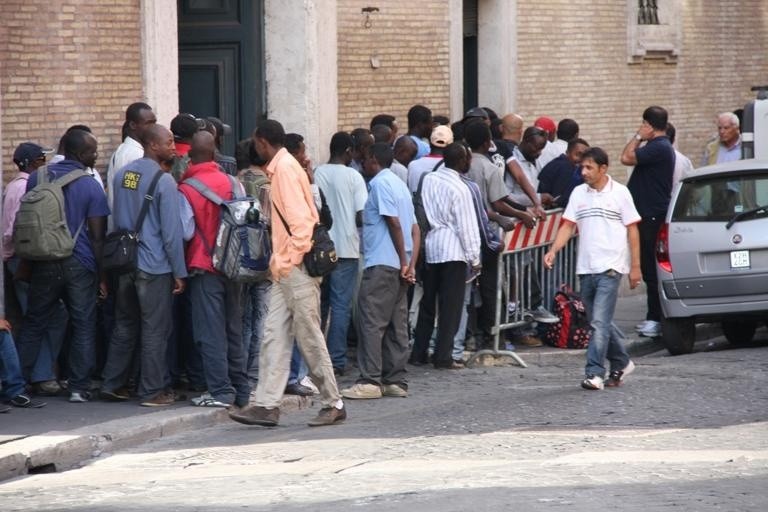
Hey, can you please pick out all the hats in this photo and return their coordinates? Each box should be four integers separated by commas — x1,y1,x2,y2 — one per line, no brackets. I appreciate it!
464,105,489,118
11,142,54,171
535,116,556,136
170,111,206,140
429,123,454,149
205,114,233,138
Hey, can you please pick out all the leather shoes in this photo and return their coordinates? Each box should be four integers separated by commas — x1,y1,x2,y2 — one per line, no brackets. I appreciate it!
407,357,423,365
445,358,465,370
307,401,347,427
229,406,280,426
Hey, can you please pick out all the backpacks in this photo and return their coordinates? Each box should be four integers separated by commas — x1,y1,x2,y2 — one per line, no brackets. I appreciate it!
182,174,273,283
11,161,93,261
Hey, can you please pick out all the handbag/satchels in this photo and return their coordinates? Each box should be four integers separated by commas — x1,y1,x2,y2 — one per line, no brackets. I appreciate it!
546,282,594,349
301,221,340,277
100,227,140,274
412,193,431,236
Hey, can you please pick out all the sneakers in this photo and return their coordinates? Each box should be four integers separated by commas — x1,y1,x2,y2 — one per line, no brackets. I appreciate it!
340,383,382,399
509,335,544,348
636,319,666,338
582,375,605,391
381,381,408,397
531,305,561,325
300,376,320,395
606,358,636,388
284,382,314,396
636,320,649,332
1,379,232,415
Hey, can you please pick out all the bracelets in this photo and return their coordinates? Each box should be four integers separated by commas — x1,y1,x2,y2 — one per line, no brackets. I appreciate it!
633,134,643,143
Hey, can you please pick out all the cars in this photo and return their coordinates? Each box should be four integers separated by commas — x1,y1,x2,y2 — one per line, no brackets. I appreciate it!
652,158,767,356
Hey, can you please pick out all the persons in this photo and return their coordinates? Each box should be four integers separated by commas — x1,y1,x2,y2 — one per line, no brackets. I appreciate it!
543,148,643,392
621,106,676,338
229,118,348,427
309,104,559,400
538,137,591,209
703,112,755,213
665,122,695,222
0,105,320,408
534,118,580,179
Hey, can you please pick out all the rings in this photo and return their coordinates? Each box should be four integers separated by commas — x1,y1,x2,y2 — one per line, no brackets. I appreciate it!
636,282,641,286
